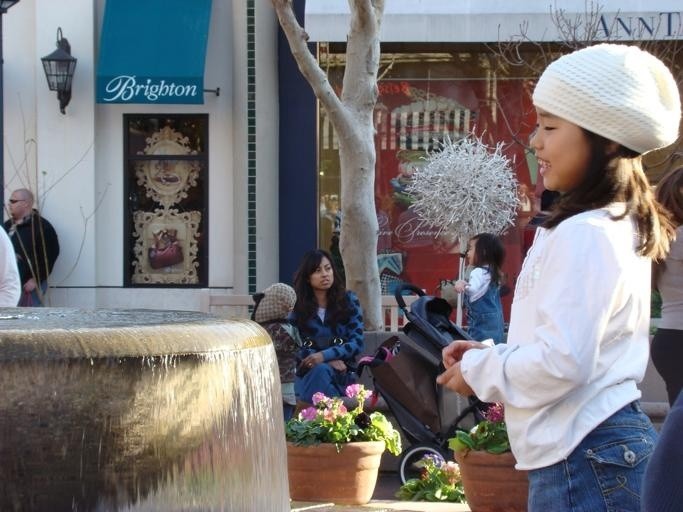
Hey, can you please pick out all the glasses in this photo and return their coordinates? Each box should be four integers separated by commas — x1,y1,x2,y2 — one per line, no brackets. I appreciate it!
10,200,25,204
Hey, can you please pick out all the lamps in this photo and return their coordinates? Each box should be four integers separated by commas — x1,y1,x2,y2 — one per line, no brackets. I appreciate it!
39,26,77,115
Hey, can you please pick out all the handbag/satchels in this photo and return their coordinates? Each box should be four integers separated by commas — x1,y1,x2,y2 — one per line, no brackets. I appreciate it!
440,279,457,308
295,338,357,376
149,241,183,269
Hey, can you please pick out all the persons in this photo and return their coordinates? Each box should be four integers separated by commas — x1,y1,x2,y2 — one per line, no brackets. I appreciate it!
374,195,393,254
521,189,561,264
454,231,505,343
650,164,683,407
390,197,434,250
251,282,304,421
328,210,345,282
434,44,683,511
2,188,61,307
287,250,365,414
0,225,24,308
638,387,682,510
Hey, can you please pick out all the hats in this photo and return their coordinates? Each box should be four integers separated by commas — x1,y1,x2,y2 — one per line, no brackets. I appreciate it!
533,43,682,155
251,284,297,322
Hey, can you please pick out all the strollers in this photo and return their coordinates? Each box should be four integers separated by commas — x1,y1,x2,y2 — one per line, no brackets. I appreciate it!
354,282,496,489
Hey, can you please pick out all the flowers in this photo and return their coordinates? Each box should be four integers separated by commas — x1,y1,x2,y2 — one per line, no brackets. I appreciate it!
394,450,467,503
448,398,518,463
288,379,406,457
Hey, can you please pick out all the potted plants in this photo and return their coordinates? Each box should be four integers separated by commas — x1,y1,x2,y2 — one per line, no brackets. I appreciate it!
453,449,528,512
281,438,385,504
